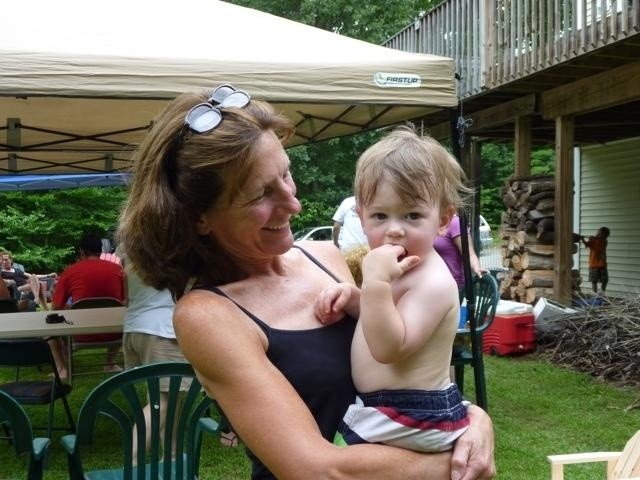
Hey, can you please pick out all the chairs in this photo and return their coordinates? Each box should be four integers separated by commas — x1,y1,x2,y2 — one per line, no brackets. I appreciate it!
546,428,640,480
449,272,499,414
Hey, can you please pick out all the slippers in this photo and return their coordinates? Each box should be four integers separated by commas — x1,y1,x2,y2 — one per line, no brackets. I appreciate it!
219,434,238,441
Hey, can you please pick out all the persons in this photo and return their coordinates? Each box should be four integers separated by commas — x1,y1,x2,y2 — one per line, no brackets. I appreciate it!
120,250,199,465
117,86,497,479
220,420,240,448
0,251,50,312
432,213,488,329
333,194,372,255
41,232,128,380
313,119,474,451
342,248,368,290
583,227,610,291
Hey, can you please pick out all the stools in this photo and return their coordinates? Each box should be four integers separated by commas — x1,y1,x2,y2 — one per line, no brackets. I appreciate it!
482,313,536,356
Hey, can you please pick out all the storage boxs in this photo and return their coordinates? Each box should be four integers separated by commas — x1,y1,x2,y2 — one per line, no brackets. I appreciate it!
532,297,578,333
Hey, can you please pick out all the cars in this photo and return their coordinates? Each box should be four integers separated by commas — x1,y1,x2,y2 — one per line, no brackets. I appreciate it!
292,225,337,244
479,215,494,253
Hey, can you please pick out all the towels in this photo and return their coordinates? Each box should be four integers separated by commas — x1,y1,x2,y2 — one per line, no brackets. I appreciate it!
474,295,533,314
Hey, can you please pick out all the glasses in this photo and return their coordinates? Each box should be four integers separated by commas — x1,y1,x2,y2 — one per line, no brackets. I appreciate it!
171,84,252,162
